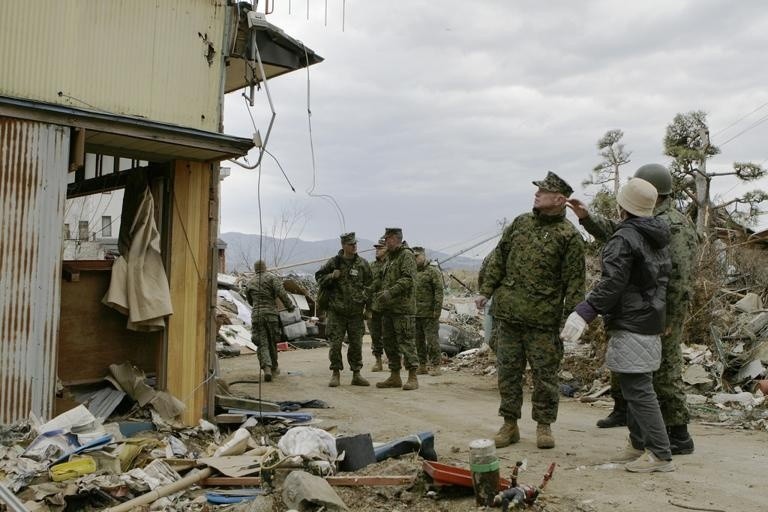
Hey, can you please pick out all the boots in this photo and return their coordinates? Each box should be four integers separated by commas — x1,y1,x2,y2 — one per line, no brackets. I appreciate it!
536,420,554,449
329,370,340,387
372,357,443,389
493,416,519,447
264,366,280,382
351,370,370,386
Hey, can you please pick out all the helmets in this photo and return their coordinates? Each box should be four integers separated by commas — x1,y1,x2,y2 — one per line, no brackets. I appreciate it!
634,163,672,196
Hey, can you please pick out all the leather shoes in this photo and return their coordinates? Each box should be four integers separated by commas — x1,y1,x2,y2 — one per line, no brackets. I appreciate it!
596,410,627,428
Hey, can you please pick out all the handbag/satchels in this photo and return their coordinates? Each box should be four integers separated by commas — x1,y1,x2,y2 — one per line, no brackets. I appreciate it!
317,286,329,310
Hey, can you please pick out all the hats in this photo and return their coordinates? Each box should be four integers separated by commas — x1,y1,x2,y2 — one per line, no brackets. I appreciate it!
410,247,426,255
253,259,266,267
371,227,402,247
532,171,574,198
339,232,359,245
615,178,658,218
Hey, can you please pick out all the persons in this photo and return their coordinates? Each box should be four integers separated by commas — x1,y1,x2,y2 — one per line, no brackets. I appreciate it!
244,260,297,381
314,227,444,388
559,177,674,471
473,170,586,449
632,163,694,456
567,194,629,429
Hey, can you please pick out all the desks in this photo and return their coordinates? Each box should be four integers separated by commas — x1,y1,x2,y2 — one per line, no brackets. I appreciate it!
56,260,160,397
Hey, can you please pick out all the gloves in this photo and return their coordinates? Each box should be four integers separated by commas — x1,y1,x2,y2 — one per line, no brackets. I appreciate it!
560,311,587,342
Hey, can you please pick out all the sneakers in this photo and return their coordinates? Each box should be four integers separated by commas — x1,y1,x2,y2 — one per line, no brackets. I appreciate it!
608,435,695,471
